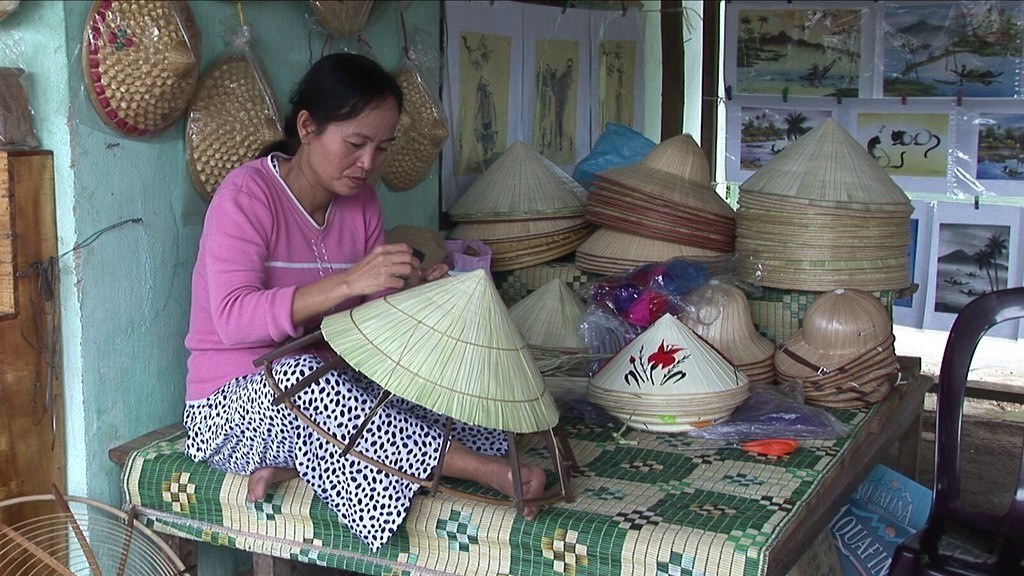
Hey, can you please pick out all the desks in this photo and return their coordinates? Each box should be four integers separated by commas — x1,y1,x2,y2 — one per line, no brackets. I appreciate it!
109,356,934,576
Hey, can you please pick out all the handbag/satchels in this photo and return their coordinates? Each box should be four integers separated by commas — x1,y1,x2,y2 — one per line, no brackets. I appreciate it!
572,122,657,192
441,239,493,279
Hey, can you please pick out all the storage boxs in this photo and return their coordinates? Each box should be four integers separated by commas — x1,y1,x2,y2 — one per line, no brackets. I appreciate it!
830,461,933,576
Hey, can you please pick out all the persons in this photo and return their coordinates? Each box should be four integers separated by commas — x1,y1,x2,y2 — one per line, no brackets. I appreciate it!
183,52,546,523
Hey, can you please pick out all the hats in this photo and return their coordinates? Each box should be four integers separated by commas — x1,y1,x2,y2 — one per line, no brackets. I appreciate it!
82,1,203,140
309,0,376,37
185,53,286,203
447,117,915,433
320,268,560,433
379,68,448,191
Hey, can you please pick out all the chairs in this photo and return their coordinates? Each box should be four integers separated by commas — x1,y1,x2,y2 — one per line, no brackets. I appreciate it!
885,287,1023,576
